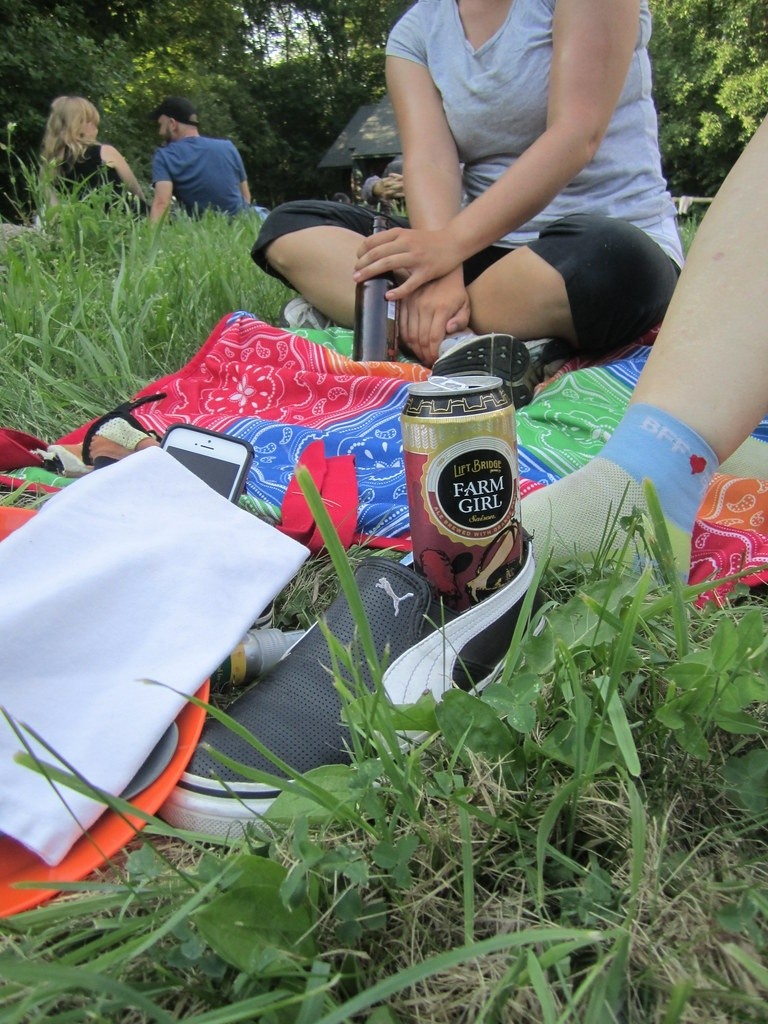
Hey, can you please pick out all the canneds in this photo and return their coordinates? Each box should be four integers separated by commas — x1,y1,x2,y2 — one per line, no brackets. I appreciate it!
401,375,524,612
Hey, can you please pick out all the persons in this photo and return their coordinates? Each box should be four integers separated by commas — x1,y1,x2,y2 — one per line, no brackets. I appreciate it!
398,112,768,592
247,1,682,408
141,96,270,248
42,94,150,223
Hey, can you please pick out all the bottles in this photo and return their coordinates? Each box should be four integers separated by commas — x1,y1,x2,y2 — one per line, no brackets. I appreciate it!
353,216,394,362
209,628,306,693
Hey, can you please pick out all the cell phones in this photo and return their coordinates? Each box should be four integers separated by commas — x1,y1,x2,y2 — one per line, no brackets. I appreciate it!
159,424,255,505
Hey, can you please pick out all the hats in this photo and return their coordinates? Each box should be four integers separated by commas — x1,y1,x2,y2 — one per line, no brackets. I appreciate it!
145,98,201,128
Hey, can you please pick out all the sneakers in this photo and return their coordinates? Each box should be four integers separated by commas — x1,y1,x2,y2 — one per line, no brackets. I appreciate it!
155,521,547,849
430,326,560,410
